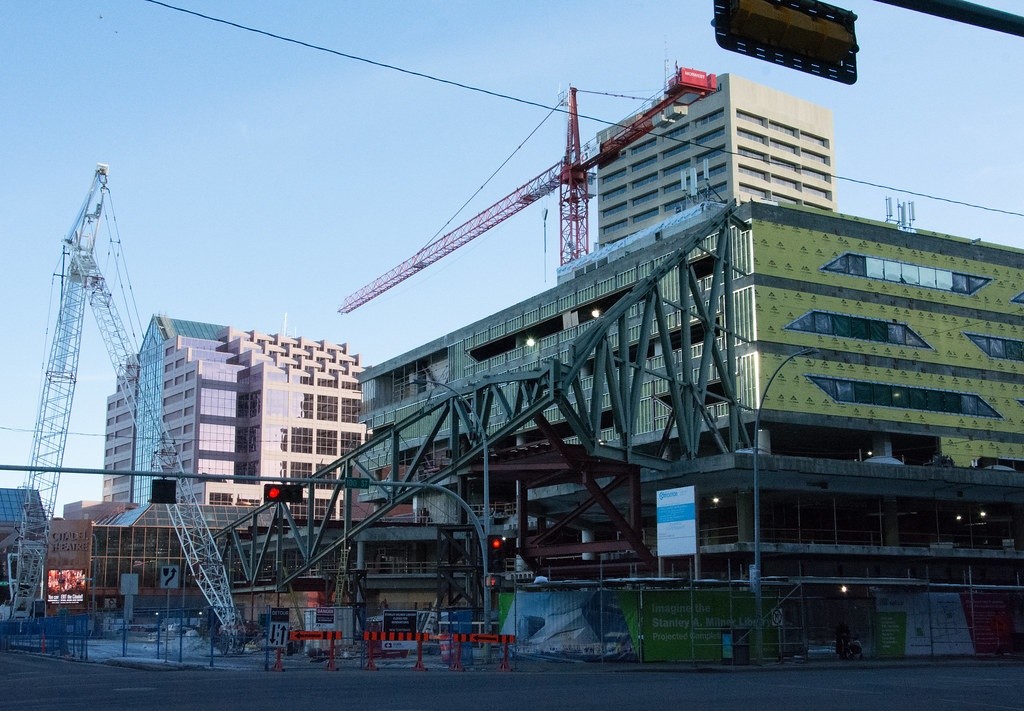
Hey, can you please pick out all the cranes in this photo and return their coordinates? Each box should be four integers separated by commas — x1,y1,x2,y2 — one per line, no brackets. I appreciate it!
5,162,249,651
336,62,716,318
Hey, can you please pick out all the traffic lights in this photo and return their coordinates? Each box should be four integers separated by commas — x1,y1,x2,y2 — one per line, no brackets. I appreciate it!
485,575,501,589
263,484,304,504
487,534,505,573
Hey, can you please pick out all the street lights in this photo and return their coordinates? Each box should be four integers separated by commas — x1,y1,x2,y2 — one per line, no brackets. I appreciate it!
750,345,820,664
